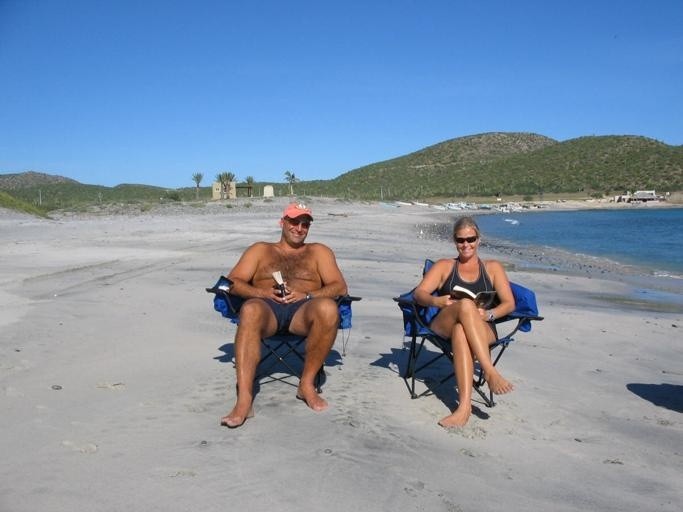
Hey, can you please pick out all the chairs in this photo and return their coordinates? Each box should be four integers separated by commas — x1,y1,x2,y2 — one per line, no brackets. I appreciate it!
209,275,361,397
393,260,543,405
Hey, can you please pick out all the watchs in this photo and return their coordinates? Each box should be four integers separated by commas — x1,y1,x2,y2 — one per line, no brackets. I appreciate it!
487,310,494,322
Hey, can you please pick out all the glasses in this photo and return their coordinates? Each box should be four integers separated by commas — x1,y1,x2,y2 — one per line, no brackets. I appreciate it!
289,219,310,229
455,235,477,244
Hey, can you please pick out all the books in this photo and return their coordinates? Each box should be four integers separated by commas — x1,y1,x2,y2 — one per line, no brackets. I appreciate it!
271,271,285,298
449,284,496,311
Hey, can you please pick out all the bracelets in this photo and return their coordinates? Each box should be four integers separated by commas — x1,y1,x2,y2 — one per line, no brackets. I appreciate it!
304,291,311,300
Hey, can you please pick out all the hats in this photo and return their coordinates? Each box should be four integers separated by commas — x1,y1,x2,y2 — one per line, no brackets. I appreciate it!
283,203,313,222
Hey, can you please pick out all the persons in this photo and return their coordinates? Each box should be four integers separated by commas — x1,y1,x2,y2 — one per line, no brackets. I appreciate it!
411,217,516,428
219,203,347,428
